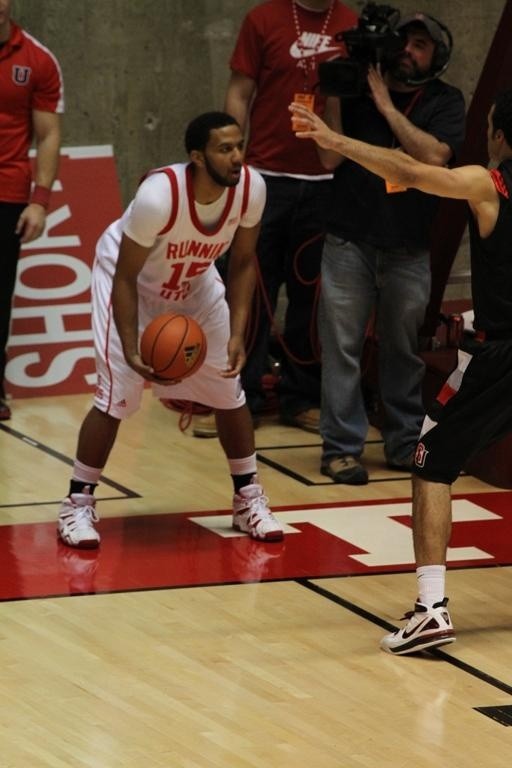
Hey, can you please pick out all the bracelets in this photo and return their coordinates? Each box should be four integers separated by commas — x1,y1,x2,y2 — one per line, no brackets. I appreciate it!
30,183,53,210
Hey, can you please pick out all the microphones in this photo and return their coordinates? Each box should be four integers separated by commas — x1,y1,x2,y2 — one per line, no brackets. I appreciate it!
405,77,432,87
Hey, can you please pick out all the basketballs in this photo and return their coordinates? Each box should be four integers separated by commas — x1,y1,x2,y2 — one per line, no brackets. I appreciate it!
140,314,208,382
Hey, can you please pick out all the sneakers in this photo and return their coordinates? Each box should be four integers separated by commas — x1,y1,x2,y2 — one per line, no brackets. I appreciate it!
320,455,368,484
381,597,456,654
233,484,282,541
285,408,322,430
58,494,100,548
194,402,259,436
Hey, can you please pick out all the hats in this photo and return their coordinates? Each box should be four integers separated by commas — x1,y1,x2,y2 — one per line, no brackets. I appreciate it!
396,13,443,42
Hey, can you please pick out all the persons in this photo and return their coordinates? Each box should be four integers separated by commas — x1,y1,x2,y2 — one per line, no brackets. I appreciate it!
56,109,283,549
287,98,511,655
0,0,65,420
317,12,469,486
55,535,287,596
191,0,362,440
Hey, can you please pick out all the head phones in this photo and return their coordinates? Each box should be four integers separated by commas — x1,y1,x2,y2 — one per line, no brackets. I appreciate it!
431,15,453,82
